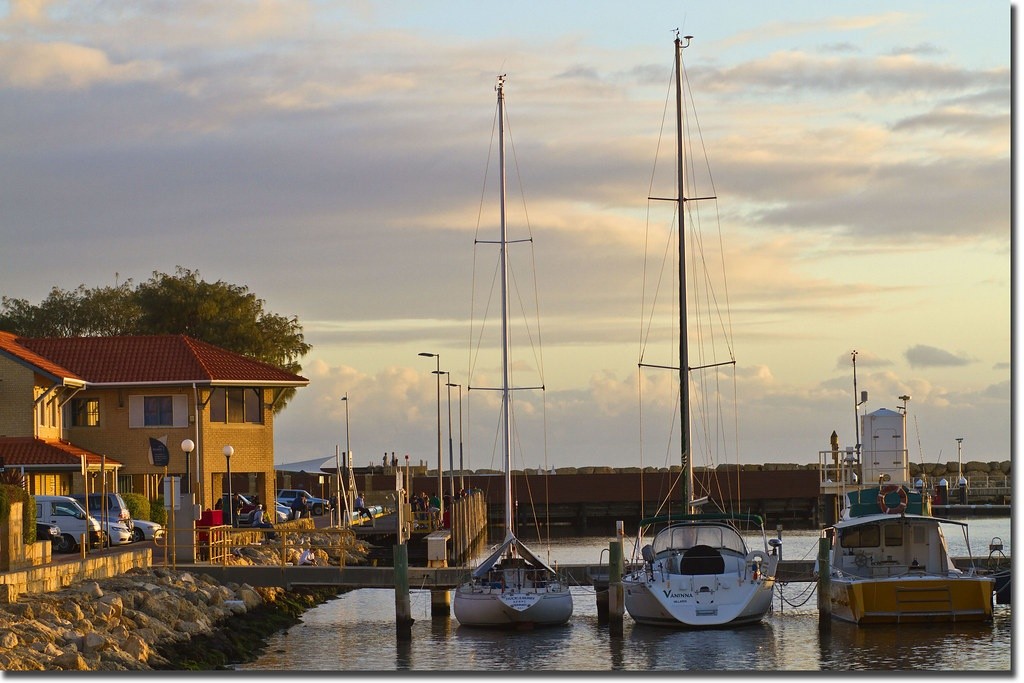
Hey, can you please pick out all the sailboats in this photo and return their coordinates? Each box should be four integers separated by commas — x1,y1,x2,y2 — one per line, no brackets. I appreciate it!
614,27,786,632
449,73,576,626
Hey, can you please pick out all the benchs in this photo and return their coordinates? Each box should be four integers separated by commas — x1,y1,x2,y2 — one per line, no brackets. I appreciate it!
346,505,383,521
231,517,315,545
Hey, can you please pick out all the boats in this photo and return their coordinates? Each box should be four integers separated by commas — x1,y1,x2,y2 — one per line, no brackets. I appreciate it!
819,347,933,529
813,503,996,631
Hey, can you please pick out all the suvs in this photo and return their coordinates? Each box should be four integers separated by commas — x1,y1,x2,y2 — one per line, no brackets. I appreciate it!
275,488,329,515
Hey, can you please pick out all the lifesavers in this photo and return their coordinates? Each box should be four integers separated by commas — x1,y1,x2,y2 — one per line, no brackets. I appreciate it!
877,485,908,515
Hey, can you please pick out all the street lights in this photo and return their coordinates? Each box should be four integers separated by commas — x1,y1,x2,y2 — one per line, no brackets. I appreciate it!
341,391,353,525
445,383,464,494
223,444,234,524
181,438,194,493
418,352,444,523
429,370,455,501
955,437,964,482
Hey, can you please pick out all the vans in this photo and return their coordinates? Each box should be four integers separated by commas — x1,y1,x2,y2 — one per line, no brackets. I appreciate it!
59,493,134,535
33,495,105,554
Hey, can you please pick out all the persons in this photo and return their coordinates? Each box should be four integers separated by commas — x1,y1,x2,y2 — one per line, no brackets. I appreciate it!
251,504,276,539
353,492,374,520
410,486,477,520
383,453,390,465
291,492,308,518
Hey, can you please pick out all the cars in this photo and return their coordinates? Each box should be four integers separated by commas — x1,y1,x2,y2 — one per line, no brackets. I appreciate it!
222,492,294,525
35,522,66,552
131,520,165,542
96,519,133,549
